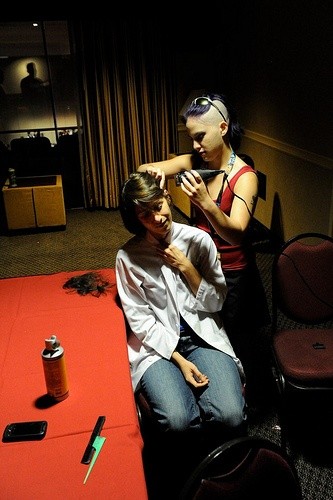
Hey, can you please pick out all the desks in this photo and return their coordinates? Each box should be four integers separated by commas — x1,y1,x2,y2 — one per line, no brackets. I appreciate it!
1,268,148,500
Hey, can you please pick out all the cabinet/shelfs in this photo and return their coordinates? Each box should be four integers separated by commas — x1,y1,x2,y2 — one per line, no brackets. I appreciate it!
1,175,67,230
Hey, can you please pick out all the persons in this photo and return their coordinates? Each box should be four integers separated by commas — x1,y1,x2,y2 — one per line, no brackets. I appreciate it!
116,173,248,480
137,91,259,370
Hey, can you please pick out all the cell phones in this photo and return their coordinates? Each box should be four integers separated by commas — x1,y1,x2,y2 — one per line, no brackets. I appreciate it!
3,421,47,442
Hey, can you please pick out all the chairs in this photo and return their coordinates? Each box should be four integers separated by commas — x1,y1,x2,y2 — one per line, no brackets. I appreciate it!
270,232,333,453
180,436,302,500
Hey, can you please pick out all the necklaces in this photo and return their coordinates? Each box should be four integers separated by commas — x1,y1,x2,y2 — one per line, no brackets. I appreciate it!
203,142,237,261
